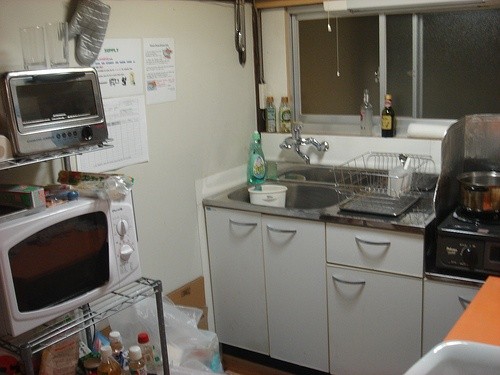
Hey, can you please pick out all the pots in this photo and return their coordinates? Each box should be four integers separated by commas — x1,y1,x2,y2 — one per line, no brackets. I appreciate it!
455,170,500,216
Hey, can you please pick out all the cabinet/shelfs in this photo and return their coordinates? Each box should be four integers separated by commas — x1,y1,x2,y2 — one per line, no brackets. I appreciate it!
323,216,436,375
0,279,171,374
423,273,485,359
202,202,331,374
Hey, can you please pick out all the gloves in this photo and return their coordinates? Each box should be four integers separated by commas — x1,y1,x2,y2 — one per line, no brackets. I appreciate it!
69,0,111,66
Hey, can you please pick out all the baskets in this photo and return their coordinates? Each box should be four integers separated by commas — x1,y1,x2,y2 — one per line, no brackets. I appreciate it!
332,151,436,215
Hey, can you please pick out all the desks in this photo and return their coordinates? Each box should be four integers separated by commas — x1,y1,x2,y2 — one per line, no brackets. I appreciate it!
441,274,499,348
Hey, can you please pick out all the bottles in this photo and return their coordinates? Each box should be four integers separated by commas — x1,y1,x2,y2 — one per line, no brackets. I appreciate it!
127,346,148,375
382,94,393,137
265,96,276,133
279,96,292,132
360,89,373,137
138,332,155,374
99,345,121,374
108,331,124,358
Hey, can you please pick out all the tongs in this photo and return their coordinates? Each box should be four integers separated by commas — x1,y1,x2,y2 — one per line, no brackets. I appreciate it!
234,0,246,53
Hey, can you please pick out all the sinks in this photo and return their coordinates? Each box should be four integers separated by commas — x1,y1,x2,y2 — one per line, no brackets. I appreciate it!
207,180,354,210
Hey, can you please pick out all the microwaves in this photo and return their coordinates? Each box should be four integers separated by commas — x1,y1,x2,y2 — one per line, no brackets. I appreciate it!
1,185,140,339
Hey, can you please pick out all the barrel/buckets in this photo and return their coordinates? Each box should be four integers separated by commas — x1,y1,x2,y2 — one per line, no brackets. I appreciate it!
246,184,288,210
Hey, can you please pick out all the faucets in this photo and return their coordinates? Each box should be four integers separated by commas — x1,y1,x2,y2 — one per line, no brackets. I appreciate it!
279,126,329,164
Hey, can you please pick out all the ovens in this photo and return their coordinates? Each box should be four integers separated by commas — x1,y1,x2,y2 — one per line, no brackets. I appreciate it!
0,68,108,158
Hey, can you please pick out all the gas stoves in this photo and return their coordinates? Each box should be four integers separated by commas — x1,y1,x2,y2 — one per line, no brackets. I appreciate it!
437,205,500,275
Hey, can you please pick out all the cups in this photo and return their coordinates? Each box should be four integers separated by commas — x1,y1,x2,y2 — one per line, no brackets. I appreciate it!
48,21,69,68
20,26,46,69
